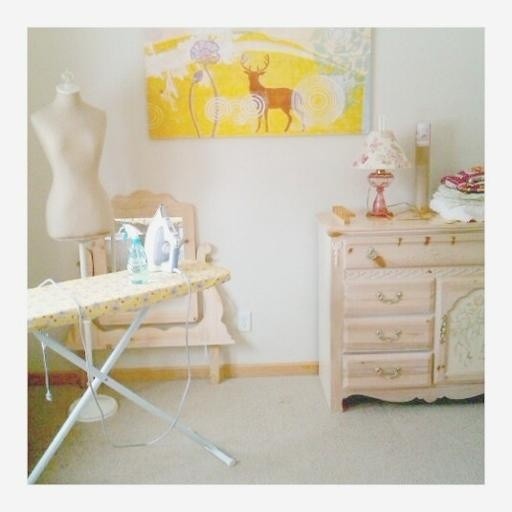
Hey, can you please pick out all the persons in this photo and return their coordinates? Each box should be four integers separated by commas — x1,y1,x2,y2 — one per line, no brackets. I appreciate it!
31,88,115,241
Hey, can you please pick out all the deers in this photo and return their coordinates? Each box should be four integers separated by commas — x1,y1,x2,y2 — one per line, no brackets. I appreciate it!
239,52,307,134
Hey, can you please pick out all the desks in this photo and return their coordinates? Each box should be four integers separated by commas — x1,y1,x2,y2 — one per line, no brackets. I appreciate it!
317,209,483,414
29,261,238,482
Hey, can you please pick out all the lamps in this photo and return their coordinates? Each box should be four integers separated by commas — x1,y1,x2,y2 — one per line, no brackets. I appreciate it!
350,129,413,221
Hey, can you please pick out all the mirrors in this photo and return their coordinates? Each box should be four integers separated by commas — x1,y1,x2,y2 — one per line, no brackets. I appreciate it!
92,188,200,326
